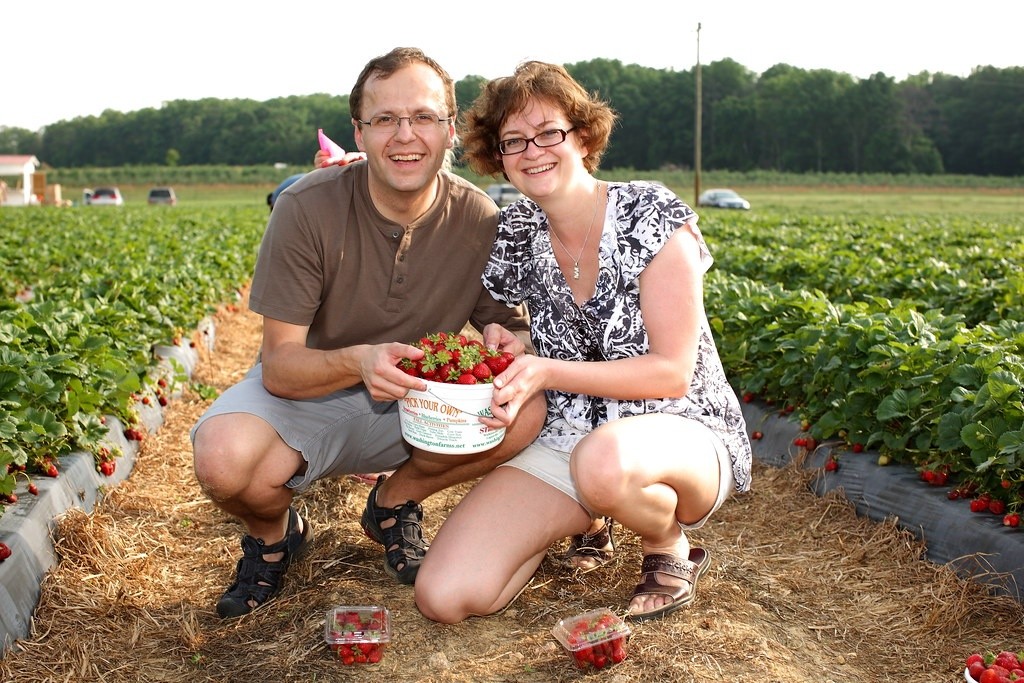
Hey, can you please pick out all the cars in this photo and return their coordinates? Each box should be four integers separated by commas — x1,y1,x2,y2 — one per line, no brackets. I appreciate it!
148,187,177,207
700,189,750,210
484,184,525,207
82,187,123,205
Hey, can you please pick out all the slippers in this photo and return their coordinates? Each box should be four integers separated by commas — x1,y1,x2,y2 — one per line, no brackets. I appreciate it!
626,546,712,620
565,515,615,573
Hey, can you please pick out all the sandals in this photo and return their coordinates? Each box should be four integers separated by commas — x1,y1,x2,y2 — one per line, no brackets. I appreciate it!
216,506,313,618
360,475,431,585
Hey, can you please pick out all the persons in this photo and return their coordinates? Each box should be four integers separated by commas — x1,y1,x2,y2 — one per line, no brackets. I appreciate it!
412,61,756,625
184,41,530,618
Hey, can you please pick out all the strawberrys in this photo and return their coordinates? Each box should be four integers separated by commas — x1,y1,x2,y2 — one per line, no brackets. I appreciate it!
561,612,626,674
740,391,1021,527
0,334,198,562
326,608,389,666
966,651,1024,683
395,331,514,385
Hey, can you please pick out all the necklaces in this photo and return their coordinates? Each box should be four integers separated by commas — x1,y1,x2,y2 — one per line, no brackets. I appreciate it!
546,181,602,281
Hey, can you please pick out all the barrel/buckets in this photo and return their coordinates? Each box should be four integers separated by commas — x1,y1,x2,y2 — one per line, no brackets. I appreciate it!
398,378,507,455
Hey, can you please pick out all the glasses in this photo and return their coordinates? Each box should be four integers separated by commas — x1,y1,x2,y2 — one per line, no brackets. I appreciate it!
495,124,579,155
358,113,451,133
